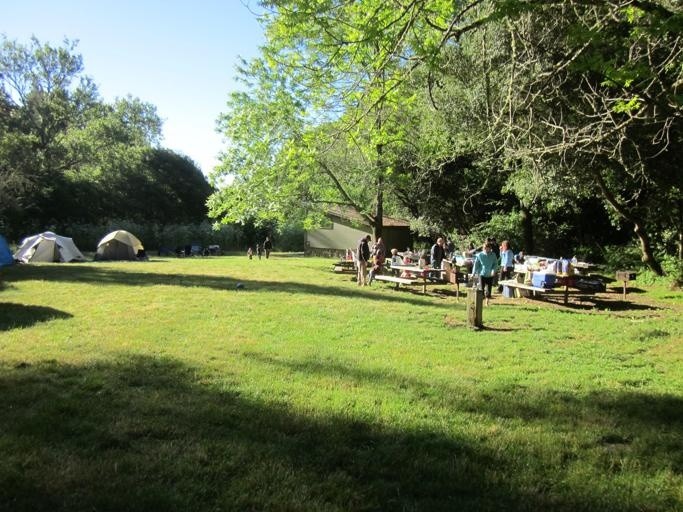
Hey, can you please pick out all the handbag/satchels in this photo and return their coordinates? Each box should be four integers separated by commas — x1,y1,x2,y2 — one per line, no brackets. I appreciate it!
524,269,532,286
418,256,425,269
546,257,571,277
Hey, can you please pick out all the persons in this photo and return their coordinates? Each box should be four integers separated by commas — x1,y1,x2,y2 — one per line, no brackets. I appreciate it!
255,243,262,260
355,234,526,308
246,247,254,261
262,237,272,259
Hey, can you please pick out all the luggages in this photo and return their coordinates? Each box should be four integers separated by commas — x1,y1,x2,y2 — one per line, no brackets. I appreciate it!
531,271,556,289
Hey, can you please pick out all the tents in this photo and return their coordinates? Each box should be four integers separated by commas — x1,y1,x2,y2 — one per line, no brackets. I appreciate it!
92,228,148,262
0,233,14,269
11,230,86,266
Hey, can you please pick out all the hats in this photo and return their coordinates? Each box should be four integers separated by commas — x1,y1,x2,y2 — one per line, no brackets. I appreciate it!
367,235,371,241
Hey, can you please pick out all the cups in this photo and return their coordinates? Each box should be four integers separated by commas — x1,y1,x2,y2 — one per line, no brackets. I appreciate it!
418,259,425,269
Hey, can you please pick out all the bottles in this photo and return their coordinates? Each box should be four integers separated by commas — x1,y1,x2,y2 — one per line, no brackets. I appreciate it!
451,255,456,268
571,256,577,265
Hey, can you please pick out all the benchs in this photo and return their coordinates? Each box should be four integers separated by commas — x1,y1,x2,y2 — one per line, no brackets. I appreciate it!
332,248,616,308
